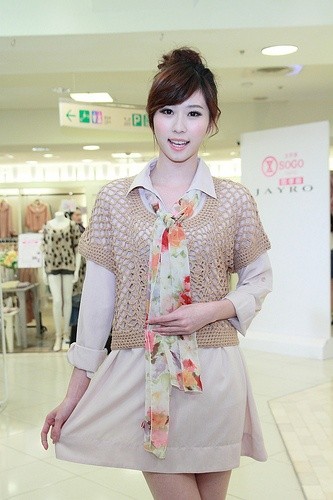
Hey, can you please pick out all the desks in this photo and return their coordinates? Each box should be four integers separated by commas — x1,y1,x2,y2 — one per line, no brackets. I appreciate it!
0,282,42,349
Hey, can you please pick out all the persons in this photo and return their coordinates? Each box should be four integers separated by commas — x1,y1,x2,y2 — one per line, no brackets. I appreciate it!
65,207,87,348
42,211,82,351
40,47,273,500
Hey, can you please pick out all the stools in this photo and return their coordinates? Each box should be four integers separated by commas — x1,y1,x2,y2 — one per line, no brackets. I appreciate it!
0,309,22,353
